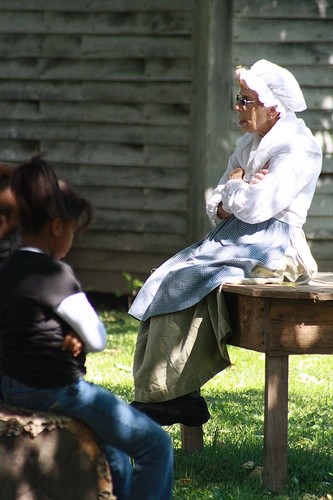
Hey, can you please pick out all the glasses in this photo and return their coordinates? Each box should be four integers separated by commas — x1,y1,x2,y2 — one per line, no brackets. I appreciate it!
235,94,261,107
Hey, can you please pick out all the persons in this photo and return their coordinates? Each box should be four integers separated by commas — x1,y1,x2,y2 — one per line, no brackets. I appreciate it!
0,157,174,500
126,58,323,426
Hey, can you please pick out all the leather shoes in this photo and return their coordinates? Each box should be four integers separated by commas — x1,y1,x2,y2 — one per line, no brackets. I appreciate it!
129,392,210,426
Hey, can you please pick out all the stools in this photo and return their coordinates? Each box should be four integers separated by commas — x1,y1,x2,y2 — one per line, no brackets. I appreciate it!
1,405,116,500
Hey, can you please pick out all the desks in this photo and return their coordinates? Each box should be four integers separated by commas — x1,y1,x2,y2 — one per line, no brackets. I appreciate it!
151,267,332,490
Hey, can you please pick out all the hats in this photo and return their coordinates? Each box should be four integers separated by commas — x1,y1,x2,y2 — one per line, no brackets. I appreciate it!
238,59,306,117
